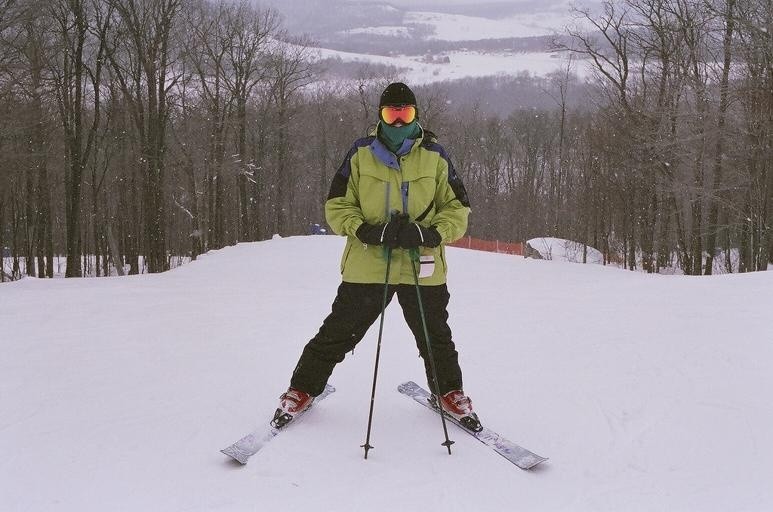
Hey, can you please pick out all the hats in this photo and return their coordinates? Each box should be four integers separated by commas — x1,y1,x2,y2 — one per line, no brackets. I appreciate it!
377,81,418,106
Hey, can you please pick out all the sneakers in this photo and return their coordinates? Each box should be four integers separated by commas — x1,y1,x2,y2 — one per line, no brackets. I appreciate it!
431,385,474,420
279,386,315,416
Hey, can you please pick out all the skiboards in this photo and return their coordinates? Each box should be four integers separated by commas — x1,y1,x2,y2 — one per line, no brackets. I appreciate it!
221,381,550,470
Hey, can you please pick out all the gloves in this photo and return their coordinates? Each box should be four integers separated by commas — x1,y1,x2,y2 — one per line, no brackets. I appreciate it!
355,221,411,249
400,222,442,248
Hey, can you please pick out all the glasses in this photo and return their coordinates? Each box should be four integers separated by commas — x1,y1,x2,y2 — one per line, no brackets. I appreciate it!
378,105,419,126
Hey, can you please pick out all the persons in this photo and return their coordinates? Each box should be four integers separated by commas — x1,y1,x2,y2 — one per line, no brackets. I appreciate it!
267,83,486,436
